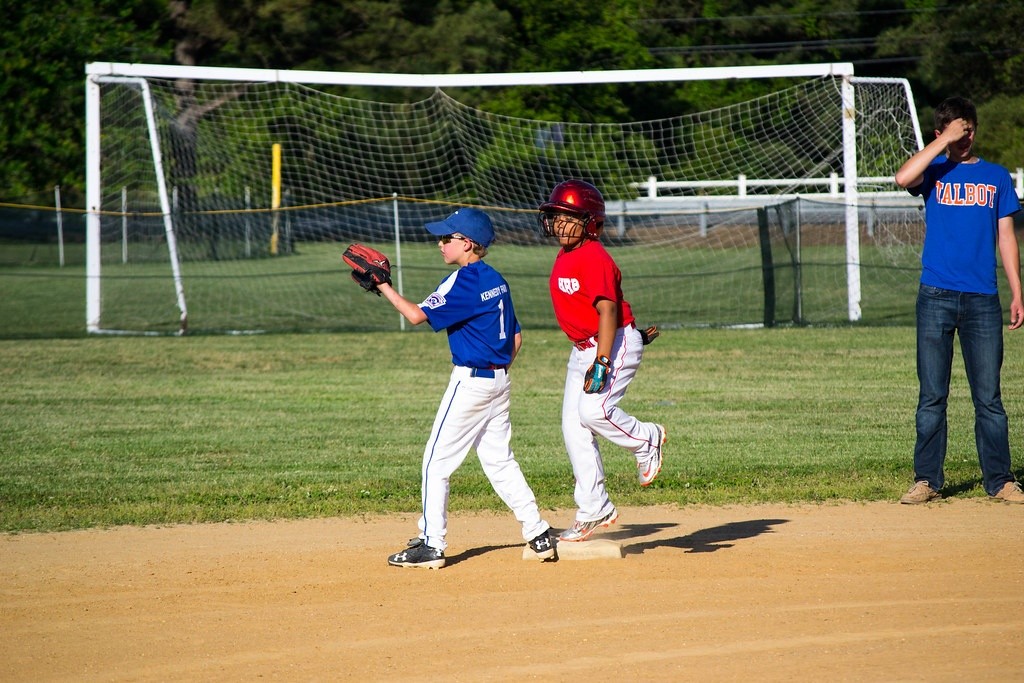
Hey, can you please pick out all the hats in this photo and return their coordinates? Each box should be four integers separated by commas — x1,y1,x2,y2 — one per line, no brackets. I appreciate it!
425,208,496,248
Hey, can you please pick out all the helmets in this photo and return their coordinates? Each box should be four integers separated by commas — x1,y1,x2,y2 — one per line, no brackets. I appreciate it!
537,180,606,239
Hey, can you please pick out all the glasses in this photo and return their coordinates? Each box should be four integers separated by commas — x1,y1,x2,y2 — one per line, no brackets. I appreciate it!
440,234,473,245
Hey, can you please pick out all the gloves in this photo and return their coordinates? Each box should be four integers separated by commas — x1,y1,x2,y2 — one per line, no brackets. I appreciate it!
636,326,659,346
583,353,612,394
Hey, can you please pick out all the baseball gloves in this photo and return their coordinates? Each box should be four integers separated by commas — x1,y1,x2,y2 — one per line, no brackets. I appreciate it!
342,244,392,294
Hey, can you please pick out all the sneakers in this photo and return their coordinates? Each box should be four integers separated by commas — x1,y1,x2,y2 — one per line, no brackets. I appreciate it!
901,480,942,504
988,481,1024,504
560,506,618,542
635,424,667,487
388,537,445,569
528,530,555,562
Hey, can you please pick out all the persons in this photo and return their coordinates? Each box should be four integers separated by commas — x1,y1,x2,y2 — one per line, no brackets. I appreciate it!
539,179,666,542
895,101,1023,504
343,208,555,569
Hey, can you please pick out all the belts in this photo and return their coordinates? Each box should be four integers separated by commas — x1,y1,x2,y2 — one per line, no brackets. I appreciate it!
470,365,507,378
574,321,636,351
